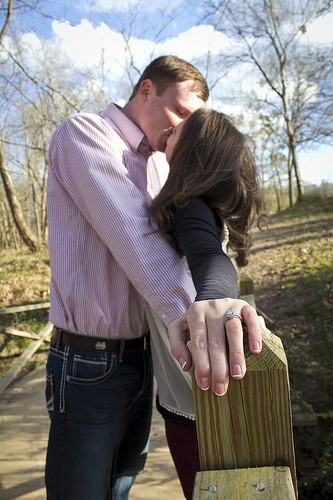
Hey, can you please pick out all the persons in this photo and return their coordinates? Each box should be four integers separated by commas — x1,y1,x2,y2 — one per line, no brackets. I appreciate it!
143,107,271,500
38,53,212,500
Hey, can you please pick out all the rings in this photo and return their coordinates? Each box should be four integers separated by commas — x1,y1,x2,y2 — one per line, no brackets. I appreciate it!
222,308,243,328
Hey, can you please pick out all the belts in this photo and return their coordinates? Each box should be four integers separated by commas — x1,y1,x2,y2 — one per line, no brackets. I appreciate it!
50,326,137,357
